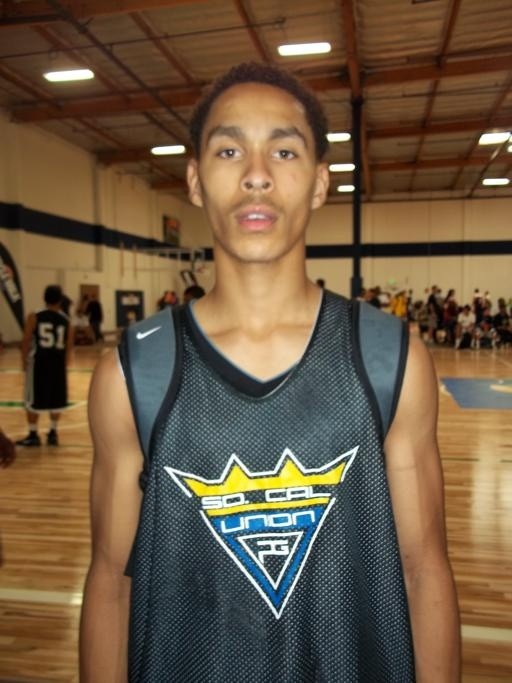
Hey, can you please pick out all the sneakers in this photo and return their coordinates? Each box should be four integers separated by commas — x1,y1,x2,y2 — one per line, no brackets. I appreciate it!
15,431,39,446
48,429,57,444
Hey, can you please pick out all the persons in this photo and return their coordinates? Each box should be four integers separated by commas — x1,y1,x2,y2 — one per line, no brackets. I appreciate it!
15,285,211,447
355,285,512,350
79,62,462,683
